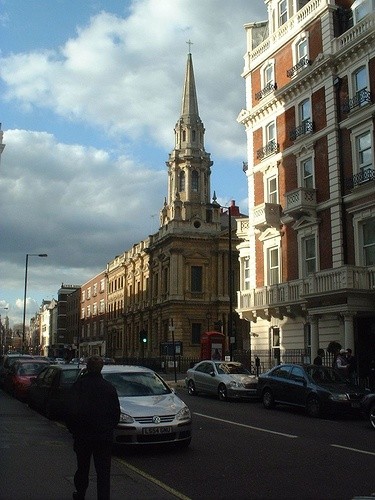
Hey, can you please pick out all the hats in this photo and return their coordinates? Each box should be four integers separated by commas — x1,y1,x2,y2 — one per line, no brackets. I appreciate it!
339,348,349,353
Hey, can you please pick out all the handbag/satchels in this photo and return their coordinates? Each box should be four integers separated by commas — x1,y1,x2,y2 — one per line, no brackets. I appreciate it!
336,358,349,377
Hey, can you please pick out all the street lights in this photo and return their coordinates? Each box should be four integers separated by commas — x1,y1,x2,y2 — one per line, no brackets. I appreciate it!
21,253,48,352
207,202,234,356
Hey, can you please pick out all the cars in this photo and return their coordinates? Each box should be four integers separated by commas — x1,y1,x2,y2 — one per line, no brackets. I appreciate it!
258,363,368,418
1,350,114,378
5,360,49,401
28,364,85,420
73,364,192,454
185,359,259,402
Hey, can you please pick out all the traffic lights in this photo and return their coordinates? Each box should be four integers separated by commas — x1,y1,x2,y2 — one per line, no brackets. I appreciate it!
140,330,147,342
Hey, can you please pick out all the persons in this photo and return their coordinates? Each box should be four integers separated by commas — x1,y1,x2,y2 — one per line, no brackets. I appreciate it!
313,349,325,367
346,349,356,378
331,349,351,377
64,354,121,500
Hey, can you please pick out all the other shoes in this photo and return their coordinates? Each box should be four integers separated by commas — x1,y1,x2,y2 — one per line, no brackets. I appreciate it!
72,488,87,500
